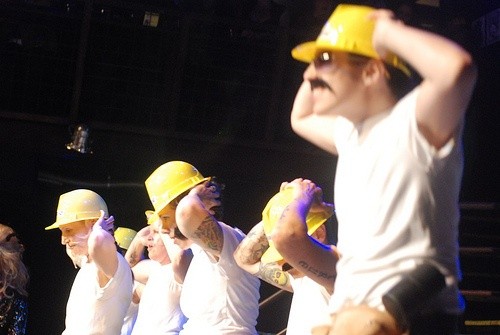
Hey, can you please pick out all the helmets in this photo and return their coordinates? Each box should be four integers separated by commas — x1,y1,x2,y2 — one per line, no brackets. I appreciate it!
261,192,335,266
291,3,412,79
113,226,137,249
143,209,158,226
45,190,109,231
144,161,211,224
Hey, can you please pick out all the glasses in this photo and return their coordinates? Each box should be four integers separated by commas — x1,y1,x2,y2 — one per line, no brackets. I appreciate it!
313,52,371,70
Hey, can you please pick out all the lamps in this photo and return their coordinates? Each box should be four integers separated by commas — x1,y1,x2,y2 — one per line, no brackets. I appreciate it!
65,123,94,154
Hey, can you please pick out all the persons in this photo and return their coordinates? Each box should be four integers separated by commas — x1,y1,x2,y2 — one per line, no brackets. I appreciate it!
145,161,261,335
234,178,342,335
0,224,30,335
113,227,146,335
125,211,188,335
291,5,476,335
45,189,134,335
